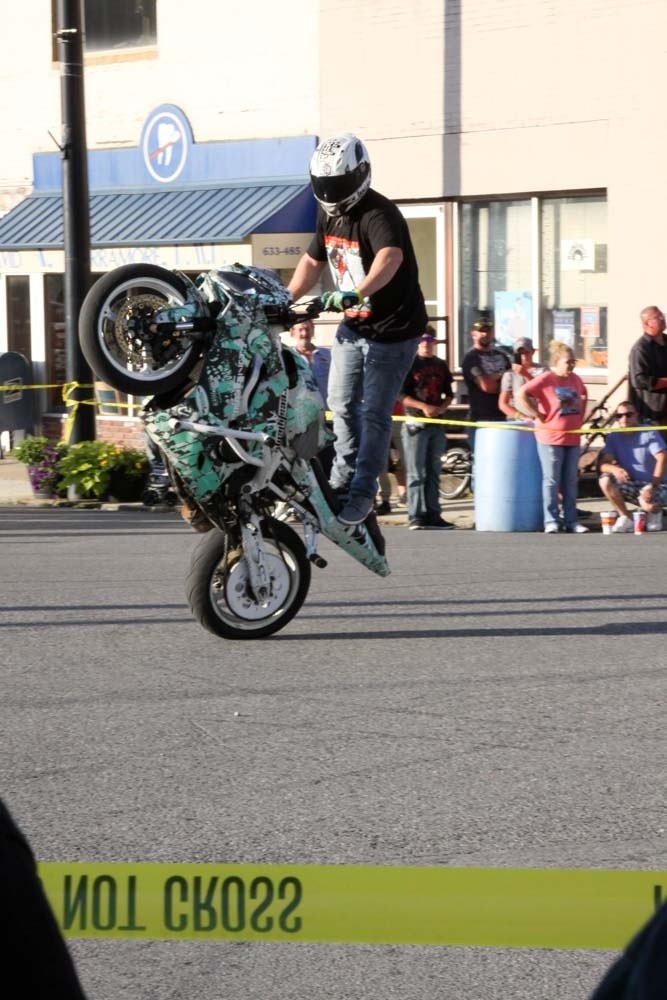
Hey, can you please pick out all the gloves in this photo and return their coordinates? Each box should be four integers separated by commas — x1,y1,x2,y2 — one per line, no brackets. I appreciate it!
320,288,364,313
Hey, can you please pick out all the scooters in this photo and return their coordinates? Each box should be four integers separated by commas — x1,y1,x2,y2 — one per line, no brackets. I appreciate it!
436,441,474,499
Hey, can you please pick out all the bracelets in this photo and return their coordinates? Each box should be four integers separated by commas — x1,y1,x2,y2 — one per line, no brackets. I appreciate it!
355,289,364,310
514,411,520,419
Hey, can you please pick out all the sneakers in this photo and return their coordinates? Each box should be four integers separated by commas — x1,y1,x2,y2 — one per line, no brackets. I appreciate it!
409,520,425,531
646,510,663,532
612,515,635,533
327,480,351,502
559,508,593,520
424,518,456,530
544,522,561,534
565,523,590,533
337,494,375,526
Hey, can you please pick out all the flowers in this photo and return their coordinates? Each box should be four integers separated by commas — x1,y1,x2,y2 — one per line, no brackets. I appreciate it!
13,436,151,497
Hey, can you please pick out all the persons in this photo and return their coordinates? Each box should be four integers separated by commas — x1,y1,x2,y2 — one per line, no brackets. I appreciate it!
599,401,667,532
499,336,549,422
627,306,667,428
286,135,429,525
461,317,512,448
519,340,590,533
396,325,455,530
289,319,332,411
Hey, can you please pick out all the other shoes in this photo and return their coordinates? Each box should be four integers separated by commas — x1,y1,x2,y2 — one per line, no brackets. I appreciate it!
395,492,408,508
373,501,391,516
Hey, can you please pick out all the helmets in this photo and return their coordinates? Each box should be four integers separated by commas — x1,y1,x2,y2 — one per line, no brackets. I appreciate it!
309,134,373,217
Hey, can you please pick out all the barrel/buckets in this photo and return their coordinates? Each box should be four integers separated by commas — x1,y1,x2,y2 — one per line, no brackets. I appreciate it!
472,421,545,531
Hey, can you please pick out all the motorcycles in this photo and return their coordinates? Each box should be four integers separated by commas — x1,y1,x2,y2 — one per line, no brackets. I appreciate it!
77,261,392,640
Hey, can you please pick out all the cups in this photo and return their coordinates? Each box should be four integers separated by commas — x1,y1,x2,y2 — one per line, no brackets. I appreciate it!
633,512,646,534
600,512,617,535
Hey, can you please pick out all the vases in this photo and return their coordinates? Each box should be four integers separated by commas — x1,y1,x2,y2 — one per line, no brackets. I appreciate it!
27,466,58,496
67,483,79,501
111,476,150,503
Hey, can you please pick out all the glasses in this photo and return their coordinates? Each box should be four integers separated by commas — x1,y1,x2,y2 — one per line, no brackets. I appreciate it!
614,411,635,419
418,335,435,344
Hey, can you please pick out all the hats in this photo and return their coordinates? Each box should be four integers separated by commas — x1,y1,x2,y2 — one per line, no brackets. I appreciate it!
513,336,532,353
472,318,495,333
